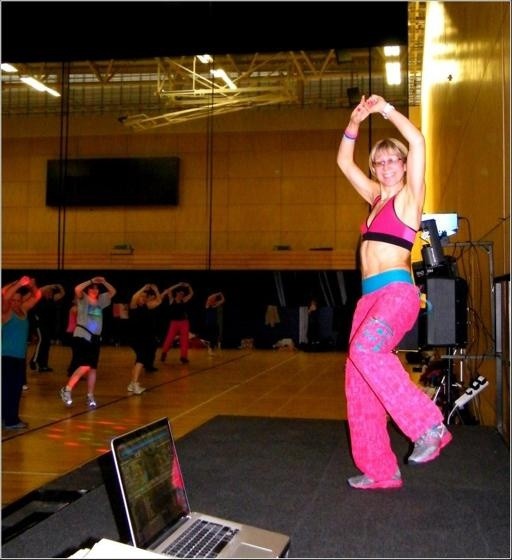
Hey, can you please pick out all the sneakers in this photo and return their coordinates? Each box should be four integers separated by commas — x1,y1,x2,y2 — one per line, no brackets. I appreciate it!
348,463,403,489
127,381,146,395
85,395,97,409
408,422,452,465
56,386,72,408
4,421,28,429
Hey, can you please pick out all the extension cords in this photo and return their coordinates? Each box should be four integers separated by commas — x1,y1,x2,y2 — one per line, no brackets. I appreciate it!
455,380,489,408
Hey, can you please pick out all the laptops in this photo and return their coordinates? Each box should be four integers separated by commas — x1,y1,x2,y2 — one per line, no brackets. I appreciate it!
111,417,291,560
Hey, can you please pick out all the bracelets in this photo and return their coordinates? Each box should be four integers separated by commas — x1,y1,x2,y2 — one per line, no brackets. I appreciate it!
343,128,357,143
382,102,395,120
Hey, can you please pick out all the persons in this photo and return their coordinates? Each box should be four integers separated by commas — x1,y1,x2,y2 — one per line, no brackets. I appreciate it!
1,276,226,431
336,94,453,489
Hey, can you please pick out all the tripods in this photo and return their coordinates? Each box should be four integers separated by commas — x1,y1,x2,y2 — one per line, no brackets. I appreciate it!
441,348,466,426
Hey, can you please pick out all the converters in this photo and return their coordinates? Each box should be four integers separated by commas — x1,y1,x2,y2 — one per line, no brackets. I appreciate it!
464,387,472,395
469,380,480,390
476,376,486,385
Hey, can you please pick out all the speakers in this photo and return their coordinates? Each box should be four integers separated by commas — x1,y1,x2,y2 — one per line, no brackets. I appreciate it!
397,276,468,350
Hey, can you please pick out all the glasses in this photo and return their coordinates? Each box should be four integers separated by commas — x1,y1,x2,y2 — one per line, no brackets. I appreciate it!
372,157,402,169
